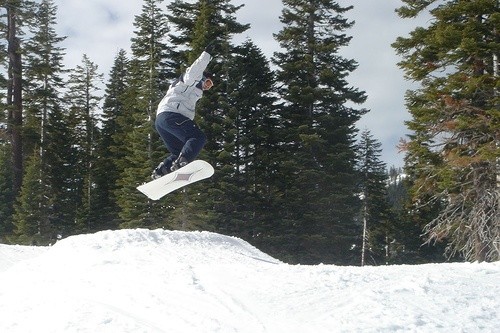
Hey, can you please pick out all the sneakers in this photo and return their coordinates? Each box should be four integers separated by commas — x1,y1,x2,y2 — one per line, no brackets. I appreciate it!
151,163,169,179
170,157,188,171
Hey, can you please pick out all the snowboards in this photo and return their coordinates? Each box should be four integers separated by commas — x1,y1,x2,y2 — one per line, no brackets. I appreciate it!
136,160,215,200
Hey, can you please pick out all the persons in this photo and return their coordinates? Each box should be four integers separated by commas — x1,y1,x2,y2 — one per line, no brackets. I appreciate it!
150,39,217,180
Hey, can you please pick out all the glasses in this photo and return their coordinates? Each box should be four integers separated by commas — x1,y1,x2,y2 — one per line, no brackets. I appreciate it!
205,79,213,90
